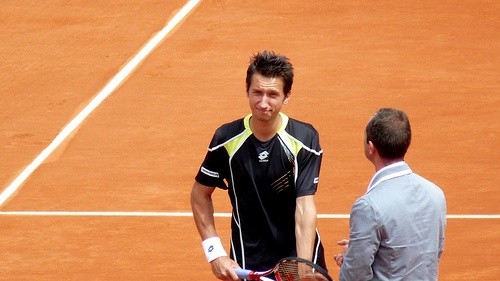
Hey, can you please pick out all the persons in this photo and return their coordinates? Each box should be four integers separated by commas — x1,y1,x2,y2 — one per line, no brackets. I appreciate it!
190,49,329,281
332,108,447,281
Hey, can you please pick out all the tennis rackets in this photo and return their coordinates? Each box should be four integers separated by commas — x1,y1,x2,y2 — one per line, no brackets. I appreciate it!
234,258,331,280
